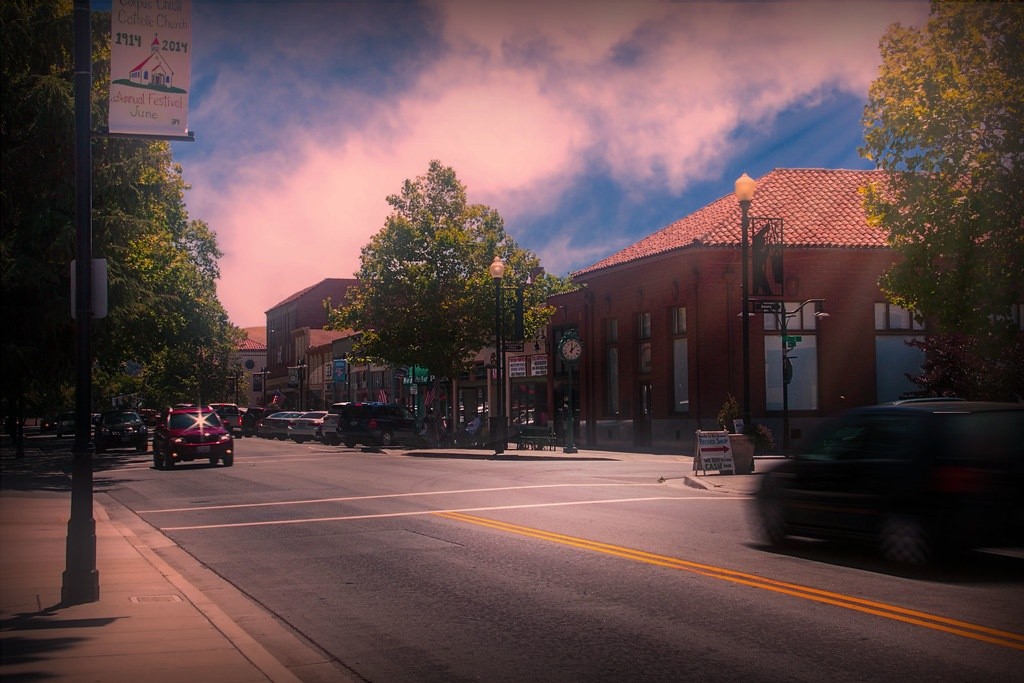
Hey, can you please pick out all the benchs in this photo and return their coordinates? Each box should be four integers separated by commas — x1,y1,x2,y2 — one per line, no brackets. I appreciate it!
516,424,557,451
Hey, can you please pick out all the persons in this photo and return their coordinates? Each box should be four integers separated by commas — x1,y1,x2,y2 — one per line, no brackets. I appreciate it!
423,410,437,449
461,411,481,448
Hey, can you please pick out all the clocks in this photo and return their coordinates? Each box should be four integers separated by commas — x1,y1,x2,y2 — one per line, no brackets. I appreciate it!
558,331,585,366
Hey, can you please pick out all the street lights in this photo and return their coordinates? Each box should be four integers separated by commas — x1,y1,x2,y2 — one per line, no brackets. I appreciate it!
733,170,756,439
343,351,353,403
233,369,246,407
260,366,271,407
296,359,308,412
489,257,506,454
737,296,830,461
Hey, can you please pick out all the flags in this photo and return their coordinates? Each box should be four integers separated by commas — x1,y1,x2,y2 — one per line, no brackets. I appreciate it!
273,389,285,411
423,375,438,409
377,386,387,403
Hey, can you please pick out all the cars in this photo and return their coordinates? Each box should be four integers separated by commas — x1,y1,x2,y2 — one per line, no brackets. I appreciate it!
260,411,306,441
286,410,330,445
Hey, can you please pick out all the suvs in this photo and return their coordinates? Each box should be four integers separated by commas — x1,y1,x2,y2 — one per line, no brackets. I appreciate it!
209,403,242,439
754,395,1023,573
40,416,58,433
152,405,233,470
58,411,76,439
322,402,353,444
336,401,419,447
95,408,147,453
242,407,282,438
